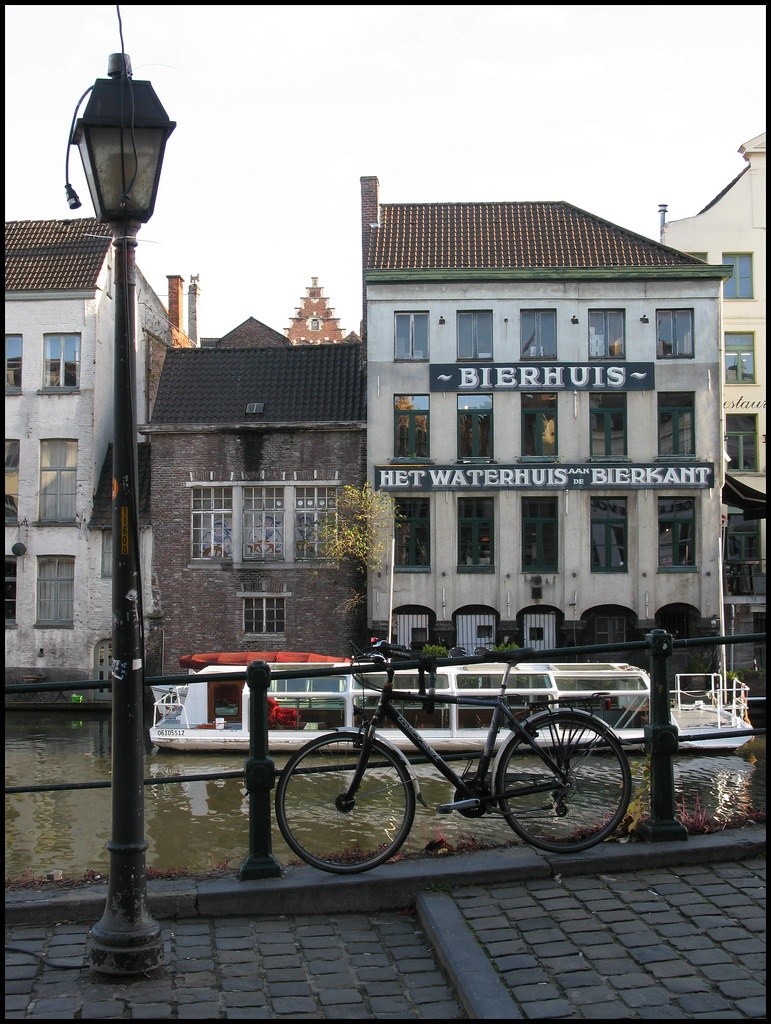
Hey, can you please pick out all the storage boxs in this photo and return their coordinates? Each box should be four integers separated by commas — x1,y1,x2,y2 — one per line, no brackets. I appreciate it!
72,694,83,703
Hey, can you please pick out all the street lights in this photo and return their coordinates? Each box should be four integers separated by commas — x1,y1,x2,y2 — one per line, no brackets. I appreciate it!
71,53,198,979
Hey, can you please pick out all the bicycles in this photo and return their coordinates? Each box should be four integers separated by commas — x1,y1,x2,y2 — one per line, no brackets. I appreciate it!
274,641,632,875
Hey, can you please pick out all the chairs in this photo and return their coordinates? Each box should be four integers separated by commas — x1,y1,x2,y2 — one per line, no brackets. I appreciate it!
10,679,30,702
55,690,70,704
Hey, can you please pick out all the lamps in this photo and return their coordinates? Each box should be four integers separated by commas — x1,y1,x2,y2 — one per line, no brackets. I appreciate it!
571,315,578,324
530,577,543,600
439,316,445,324
640,314,649,324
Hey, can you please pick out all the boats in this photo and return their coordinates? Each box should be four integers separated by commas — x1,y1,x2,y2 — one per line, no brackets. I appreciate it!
148,660,755,756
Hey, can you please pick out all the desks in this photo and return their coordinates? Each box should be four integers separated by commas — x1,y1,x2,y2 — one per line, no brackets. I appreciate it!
21,675,48,702
